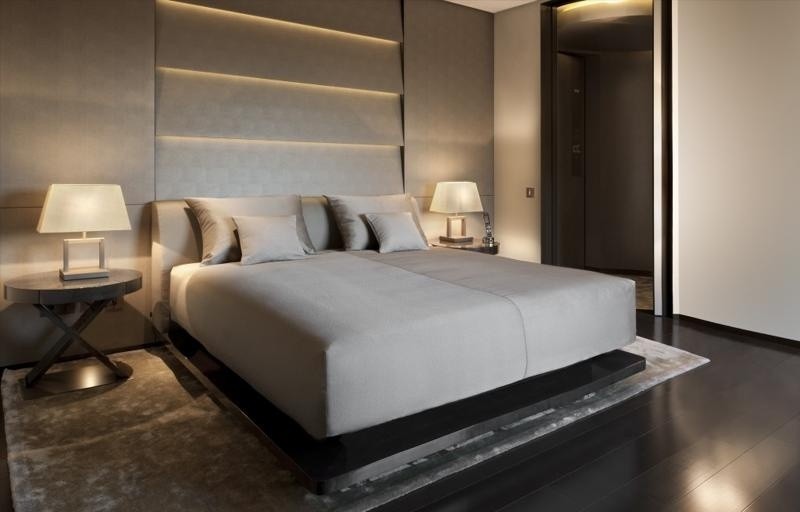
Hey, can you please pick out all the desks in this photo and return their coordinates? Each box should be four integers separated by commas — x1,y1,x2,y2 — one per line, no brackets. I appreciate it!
432,239,499,255
4,268,143,401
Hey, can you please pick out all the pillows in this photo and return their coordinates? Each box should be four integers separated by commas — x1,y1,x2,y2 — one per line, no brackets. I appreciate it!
232,215,305,265
184,194,316,266
322,193,429,250
364,212,430,254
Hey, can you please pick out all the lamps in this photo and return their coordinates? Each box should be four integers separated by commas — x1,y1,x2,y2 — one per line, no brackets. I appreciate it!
37,183,129,280
429,182,484,243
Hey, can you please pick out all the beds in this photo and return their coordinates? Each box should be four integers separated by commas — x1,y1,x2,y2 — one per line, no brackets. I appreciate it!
150,196,646,495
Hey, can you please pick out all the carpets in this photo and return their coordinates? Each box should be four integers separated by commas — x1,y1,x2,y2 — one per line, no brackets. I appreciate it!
0,337,709,512
